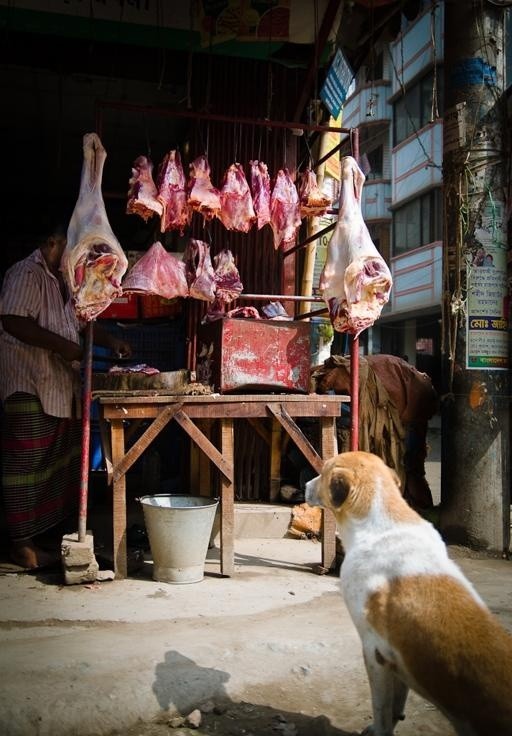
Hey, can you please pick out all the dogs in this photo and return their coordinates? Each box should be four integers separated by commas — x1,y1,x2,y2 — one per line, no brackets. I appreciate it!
304,450,512,736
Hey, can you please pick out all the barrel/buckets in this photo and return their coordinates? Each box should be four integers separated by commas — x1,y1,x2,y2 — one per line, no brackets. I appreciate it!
135,493,220,584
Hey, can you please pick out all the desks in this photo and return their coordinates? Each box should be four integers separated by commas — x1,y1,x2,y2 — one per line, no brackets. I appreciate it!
95,393,352,583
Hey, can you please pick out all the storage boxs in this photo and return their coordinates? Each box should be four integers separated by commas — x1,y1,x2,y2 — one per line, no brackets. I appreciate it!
96,289,183,321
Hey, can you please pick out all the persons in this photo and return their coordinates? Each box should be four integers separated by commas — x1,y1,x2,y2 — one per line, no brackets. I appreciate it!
2,222,134,567
